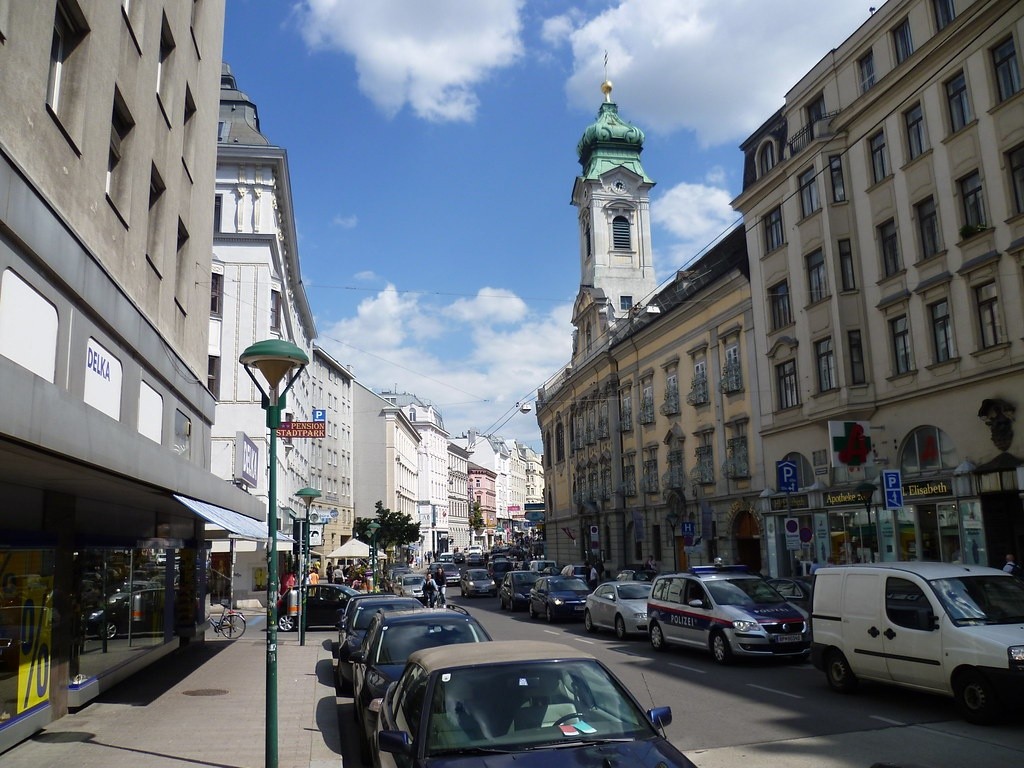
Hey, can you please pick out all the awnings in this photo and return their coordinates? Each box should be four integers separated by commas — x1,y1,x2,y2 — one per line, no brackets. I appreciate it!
176,494,296,544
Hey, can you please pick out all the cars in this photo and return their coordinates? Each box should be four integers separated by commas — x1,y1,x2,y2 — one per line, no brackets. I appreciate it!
460,569,499,599
397,574,428,598
335,594,433,697
583,580,654,640
1,541,214,676
277,583,363,632
369,640,697,768
381,562,418,596
559,565,588,584
755,574,814,616
529,576,594,625
499,570,543,613
426,545,514,586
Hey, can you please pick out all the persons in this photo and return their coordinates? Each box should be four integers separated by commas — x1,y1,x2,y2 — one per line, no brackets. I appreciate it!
410,555,421,570
825,557,834,568
424,551,433,564
484,553,489,567
313,560,320,573
584,559,600,591
647,555,656,570
421,573,438,608
809,558,822,575
326,562,333,583
333,566,345,584
434,567,447,604
280,566,319,596
1002,555,1021,584
350,573,367,594
971,540,980,564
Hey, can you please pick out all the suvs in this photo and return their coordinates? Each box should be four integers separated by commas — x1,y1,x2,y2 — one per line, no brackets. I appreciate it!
646,558,813,666
346,603,494,768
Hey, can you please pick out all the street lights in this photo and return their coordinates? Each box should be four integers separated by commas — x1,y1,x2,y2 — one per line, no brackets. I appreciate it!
294,487,322,646
367,522,381,595
238,338,310,768
519,531,525,561
666,510,680,571
854,479,878,564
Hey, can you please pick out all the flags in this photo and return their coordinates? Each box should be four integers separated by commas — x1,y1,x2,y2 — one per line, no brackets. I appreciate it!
562,527,575,540
632,511,644,541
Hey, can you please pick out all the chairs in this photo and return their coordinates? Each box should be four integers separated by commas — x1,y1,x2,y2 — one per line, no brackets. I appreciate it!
429,674,491,746
508,667,582,729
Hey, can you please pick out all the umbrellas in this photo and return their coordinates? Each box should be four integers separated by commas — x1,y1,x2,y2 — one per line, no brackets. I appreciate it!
326,539,388,559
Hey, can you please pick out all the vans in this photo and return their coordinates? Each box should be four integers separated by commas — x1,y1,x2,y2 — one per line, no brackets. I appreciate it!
530,560,558,576
810,561,1024,726
337,558,359,577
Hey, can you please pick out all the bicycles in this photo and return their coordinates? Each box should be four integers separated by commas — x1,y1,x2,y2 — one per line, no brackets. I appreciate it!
209,602,247,639
